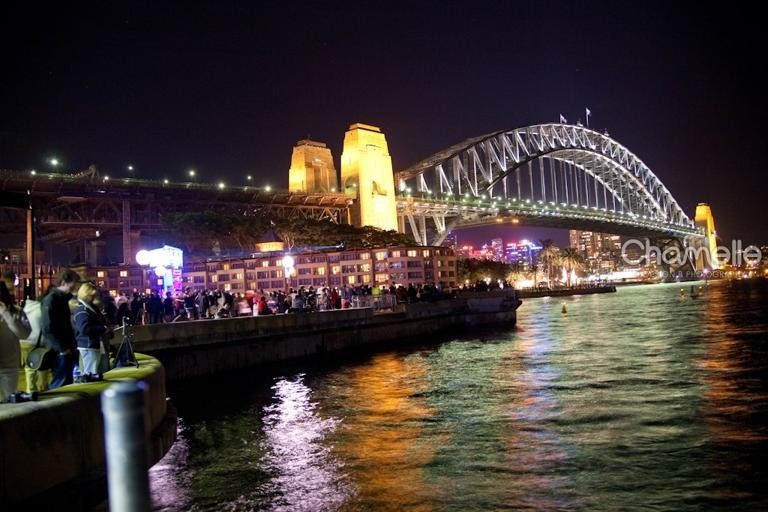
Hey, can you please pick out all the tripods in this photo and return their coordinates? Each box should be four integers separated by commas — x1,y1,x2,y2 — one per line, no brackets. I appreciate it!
112,336,139,368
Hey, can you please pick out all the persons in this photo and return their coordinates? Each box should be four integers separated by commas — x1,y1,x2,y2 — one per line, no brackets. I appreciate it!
1,277,516,402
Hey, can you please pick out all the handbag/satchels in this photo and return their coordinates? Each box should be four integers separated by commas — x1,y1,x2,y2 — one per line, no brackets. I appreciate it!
27,347,57,370
